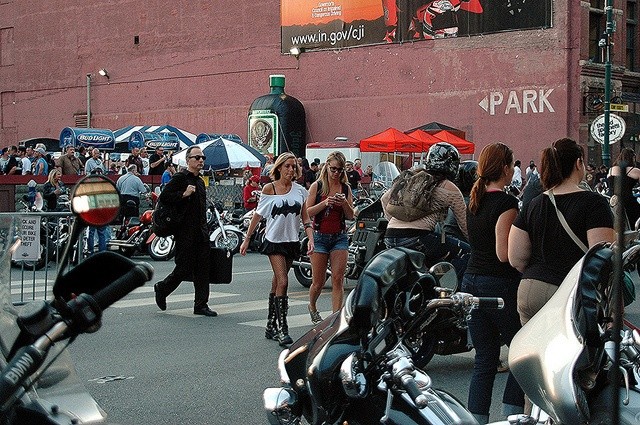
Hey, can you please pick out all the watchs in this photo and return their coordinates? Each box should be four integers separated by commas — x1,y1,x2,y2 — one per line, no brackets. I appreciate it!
291,194,386,289
506,243,639,425
1,188,77,271
204,196,267,257
345,179,389,263
0,172,155,424
260,243,640,425
107,209,177,262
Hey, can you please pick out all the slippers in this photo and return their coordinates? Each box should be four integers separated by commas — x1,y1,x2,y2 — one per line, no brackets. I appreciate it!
386,168,448,223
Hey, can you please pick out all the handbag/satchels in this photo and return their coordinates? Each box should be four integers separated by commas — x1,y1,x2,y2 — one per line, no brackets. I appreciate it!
244,234,251,239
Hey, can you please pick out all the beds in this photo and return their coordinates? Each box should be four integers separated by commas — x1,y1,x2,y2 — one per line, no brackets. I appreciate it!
360,127,430,167
402,129,444,168
432,129,475,161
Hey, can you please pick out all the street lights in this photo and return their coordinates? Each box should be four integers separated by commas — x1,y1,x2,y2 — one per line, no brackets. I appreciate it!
183,247,233,284
547,188,635,307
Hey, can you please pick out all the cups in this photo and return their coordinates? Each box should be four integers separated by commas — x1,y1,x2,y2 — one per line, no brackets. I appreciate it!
328,163,344,174
189,154,206,161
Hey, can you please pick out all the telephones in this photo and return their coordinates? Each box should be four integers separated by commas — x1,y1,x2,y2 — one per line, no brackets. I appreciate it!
609,103,628,112
590,114,626,145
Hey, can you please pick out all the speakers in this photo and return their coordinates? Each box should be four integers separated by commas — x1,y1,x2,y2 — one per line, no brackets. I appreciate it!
265,292,280,341
274,295,294,348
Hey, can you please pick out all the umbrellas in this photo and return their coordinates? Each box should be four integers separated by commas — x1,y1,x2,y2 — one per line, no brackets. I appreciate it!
154,282,167,311
193,306,217,317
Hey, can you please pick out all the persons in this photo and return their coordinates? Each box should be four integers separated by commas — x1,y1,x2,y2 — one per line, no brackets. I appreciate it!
0,143,177,186
605,148,640,230
85,167,109,256
466,142,527,425
381,143,472,278
239,151,316,347
116,164,147,222
507,139,617,420
41,170,69,214
153,145,219,317
244,176,261,250
242,155,381,195
511,160,616,186
306,150,354,325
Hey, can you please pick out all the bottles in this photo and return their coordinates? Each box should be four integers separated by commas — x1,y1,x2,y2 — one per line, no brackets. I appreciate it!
249,175,261,182
345,161,354,165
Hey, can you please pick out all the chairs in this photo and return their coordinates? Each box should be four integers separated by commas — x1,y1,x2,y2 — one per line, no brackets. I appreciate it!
307,305,323,325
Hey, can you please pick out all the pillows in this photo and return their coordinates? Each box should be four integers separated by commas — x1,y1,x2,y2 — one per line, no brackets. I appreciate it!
459,160,478,186
425,142,462,182
